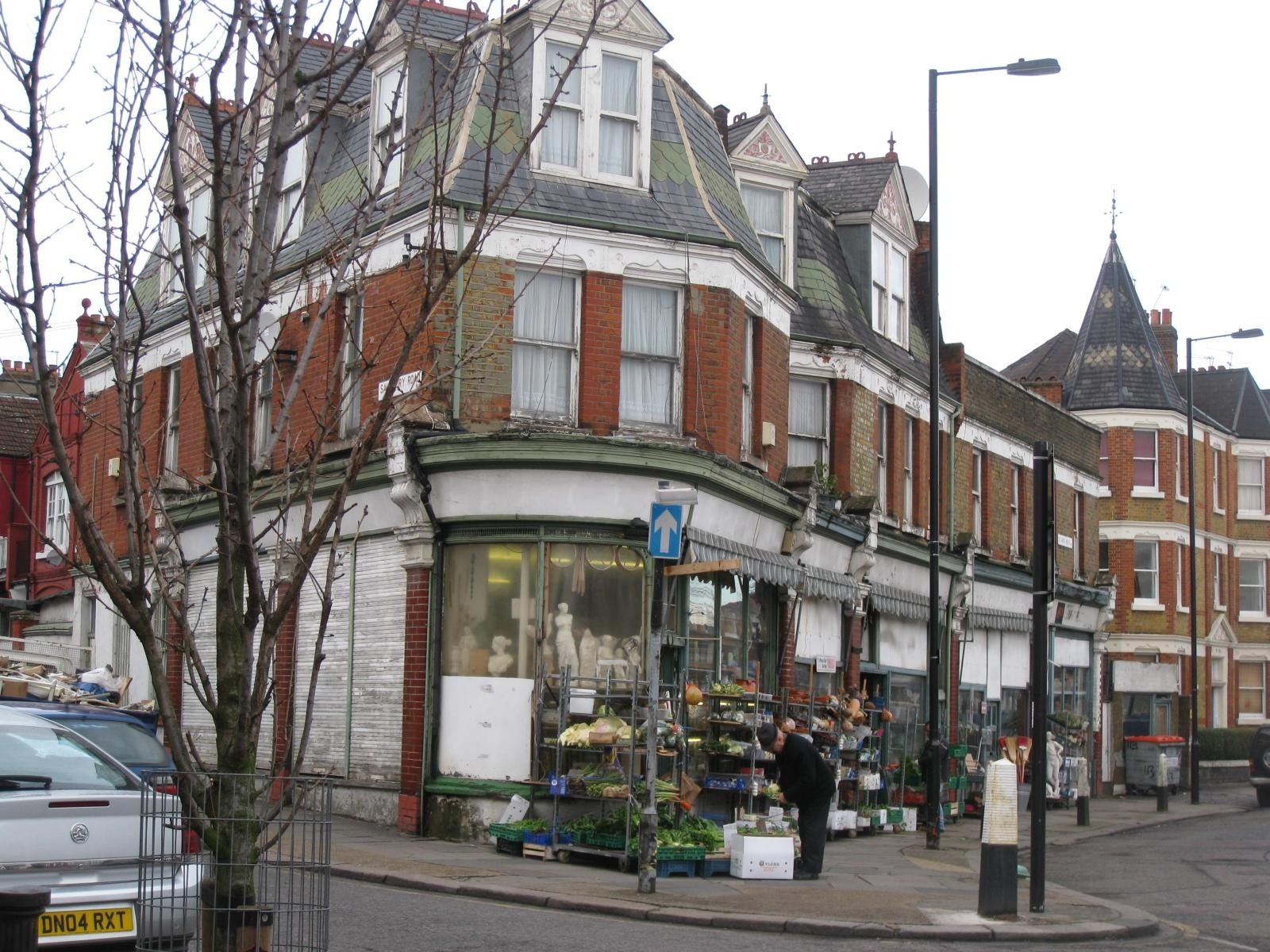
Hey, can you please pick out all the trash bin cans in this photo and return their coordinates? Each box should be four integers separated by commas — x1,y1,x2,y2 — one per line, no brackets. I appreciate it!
1124,736,1186,795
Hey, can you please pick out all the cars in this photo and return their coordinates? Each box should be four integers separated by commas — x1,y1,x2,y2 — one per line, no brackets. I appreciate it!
0,704,217,952
0,700,183,796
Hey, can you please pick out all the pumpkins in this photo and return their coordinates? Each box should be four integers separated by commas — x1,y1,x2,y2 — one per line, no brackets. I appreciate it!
842,699,860,731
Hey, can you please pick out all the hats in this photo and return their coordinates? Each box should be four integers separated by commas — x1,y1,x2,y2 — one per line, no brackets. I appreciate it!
757,722,778,751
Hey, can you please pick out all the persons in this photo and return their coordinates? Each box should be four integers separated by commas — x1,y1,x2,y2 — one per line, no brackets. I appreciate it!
915,721,949,833
458,602,643,690
757,722,836,880
1046,731,1064,796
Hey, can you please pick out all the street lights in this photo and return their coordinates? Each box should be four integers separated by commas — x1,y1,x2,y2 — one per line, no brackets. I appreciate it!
923,56,1061,847
1185,328,1264,804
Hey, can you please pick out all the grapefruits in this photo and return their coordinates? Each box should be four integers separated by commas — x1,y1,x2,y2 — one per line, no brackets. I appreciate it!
684,685,703,705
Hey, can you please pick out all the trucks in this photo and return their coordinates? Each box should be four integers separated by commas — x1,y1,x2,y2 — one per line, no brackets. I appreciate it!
0,634,93,703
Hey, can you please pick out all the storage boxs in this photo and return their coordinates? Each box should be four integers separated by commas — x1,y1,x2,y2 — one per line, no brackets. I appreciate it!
490,688,967,883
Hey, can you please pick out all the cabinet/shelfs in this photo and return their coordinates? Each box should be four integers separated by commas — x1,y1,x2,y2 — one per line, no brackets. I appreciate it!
529,663,919,869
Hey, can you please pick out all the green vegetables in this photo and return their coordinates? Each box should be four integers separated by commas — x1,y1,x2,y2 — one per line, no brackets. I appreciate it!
710,680,745,694
899,756,954,778
856,801,889,818
506,764,724,850
698,736,744,755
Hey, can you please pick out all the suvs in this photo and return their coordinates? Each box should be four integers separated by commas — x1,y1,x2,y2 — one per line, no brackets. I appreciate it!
1248,723,1270,809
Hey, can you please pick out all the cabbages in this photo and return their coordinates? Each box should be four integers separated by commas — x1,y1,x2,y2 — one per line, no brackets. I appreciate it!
557,706,641,748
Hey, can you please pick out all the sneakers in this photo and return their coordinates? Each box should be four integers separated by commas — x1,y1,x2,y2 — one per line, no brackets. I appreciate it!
794,869,819,880
